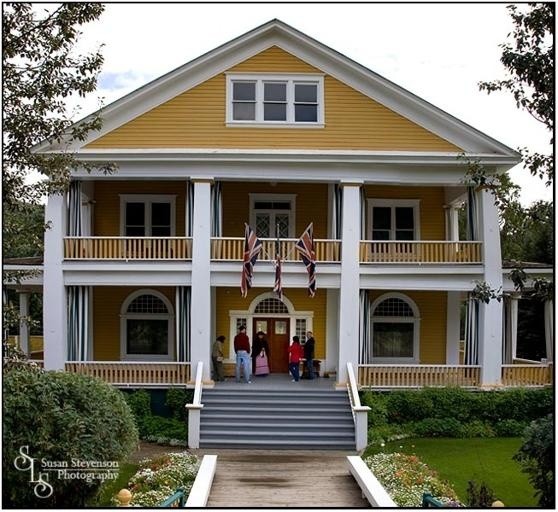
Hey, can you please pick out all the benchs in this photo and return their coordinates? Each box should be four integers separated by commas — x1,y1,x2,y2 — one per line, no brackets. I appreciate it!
241,226,262,298
272,254,282,299
295,222,316,299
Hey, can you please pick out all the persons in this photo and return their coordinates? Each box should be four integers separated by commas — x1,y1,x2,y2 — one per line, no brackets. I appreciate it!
211,336,225,381
287,336,304,383
234,326,251,383
251,331,269,378
303,332,315,379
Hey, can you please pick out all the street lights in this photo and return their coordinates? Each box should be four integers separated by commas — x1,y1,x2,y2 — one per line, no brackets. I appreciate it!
217,357,223,361
255,356,270,375
